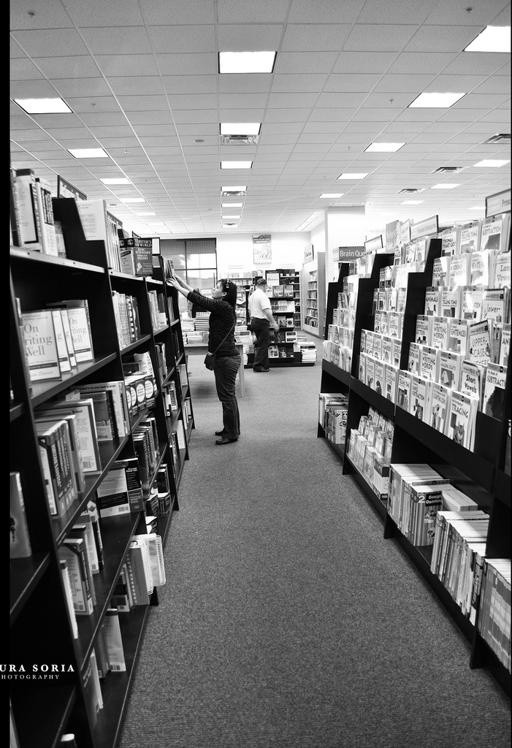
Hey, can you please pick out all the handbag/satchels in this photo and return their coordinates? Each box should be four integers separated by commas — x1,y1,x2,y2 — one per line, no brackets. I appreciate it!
204,351,216,370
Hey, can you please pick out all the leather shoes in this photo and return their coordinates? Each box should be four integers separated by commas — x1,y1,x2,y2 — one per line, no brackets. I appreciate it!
254,367,269,372
216,437,237,444
215,431,225,436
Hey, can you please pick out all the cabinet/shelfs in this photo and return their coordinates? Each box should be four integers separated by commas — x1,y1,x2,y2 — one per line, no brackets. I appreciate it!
316,213,512,700
10,199,195,748
227,269,326,368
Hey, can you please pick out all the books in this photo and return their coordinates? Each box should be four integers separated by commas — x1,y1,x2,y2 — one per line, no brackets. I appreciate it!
234,210,512,673
8,164,211,748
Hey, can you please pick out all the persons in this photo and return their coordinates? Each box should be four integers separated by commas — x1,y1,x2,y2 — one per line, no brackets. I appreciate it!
169,270,241,445
